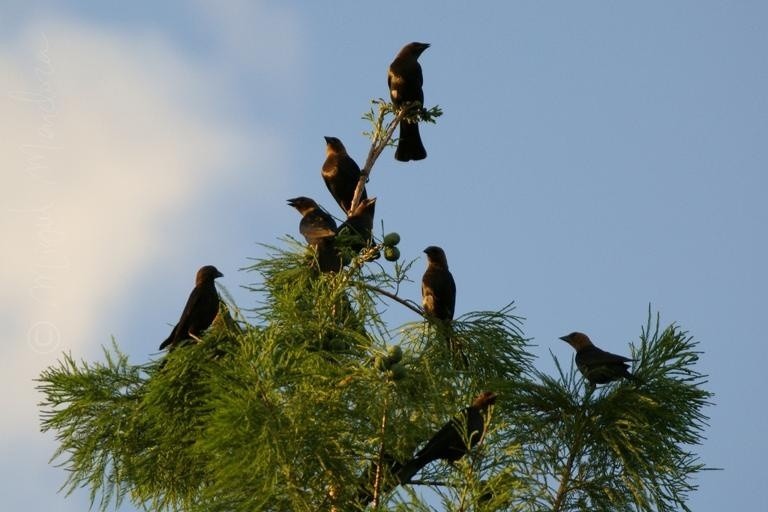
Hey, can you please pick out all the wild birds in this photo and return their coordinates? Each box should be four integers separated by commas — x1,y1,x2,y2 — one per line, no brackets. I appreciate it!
558,331,649,387
421,245,456,350
387,41,432,162
337,197,381,261
285,196,340,273
396,391,502,487
158,264,224,370
321,135,366,214
